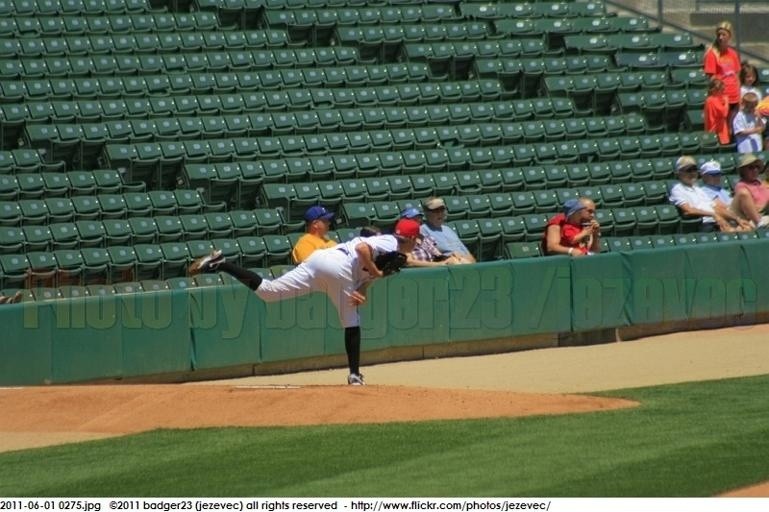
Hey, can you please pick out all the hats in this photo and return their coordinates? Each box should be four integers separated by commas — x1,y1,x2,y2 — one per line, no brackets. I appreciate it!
422,196,447,212
395,209,425,240
734,154,765,171
304,206,334,223
676,156,697,172
563,199,587,223
699,161,726,175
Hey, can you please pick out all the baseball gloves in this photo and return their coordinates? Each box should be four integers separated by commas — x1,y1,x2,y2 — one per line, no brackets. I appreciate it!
375,251,406,275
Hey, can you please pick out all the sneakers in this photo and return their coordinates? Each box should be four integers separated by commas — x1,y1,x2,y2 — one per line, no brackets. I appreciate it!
348,371,365,386
188,248,226,275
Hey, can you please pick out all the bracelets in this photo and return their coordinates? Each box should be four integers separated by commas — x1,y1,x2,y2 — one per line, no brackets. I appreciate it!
568,247,573,256
594,233,601,238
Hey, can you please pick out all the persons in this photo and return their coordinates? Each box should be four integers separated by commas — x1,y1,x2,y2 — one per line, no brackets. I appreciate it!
668,20,769,233
399,207,462,267
420,198,476,264
290,205,339,266
188,219,420,386
542,198,601,256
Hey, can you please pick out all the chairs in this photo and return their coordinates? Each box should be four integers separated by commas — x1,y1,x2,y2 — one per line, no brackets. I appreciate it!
1,1,768,298
